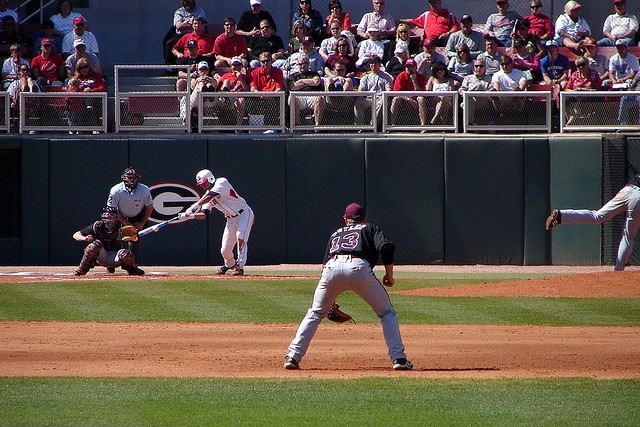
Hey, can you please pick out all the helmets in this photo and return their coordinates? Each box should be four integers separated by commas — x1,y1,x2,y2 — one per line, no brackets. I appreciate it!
101,211,117,231
196,169,215,187
121,168,139,188
496,0,508,4
601,79,613,88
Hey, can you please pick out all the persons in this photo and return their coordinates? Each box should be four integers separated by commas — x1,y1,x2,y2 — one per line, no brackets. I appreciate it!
491,54,528,111
211,17,250,67
390,58,427,134
33,20,62,56
235,0,277,37
323,38,357,77
447,43,476,82
508,38,540,69
49,0,82,35
618,70,640,125
165,0,206,66
577,37,609,81
523,0,553,39
283,36,325,76
394,23,411,62
554,0,592,51
293,0,323,38
354,24,385,67
6,64,43,107
325,60,353,110
176,40,209,91
0,0,19,32
288,20,305,55
563,56,601,125
107,167,154,231
322,0,351,38
486,0,525,47
172,17,216,58
64,79,85,135
72,57,107,134
31,38,65,86
190,61,219,109
1,43,30,90
73,207,145,276
518,18,546,59
445,14,486,63
284,202,413,371
414,38,450,77
178,169,254,276
61,16,100,59
216,56,249,134
426,61,455,124
287,57,325,133
354,55,393,125
250,51,284,92
597,0,639,46
318,19,354,61
540,40,570,93
356,0,397,40
398,0,461,47
476,36,509,75
546,174,640,271
609,39,639,84
247,19,285,69
0,15,22,45
458,58,492,111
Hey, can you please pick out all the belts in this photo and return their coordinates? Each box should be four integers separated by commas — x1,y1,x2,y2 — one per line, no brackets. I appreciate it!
227,209,244,218
332,254,359,258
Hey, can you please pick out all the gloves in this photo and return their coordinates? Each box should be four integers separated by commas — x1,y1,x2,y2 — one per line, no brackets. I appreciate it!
178,213,195,221
186,204,201,214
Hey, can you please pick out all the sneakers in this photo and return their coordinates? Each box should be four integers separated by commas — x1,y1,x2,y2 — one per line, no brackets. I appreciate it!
76,131,82,135
28,130,37,134
127,267,144,275
68,130,74,134
217,265,236,274
92,130,99,134
284,356,298,369
545,209,561,230
393,358,413,370
230,269,243,275
75,265,89,275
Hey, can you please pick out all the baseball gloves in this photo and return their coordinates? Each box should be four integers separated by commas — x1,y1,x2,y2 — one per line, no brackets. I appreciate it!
119,226,138,242
326,301,351,323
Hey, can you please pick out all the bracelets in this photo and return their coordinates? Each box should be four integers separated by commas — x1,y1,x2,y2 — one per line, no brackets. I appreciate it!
185,207,192,214
189,214,195,219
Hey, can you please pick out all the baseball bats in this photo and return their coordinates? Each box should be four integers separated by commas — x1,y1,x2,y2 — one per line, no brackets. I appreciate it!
138,216,179,237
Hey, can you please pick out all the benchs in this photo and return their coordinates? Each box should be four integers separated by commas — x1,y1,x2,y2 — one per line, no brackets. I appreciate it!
1,85,104,114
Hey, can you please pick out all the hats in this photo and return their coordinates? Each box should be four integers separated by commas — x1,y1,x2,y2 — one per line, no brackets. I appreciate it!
301,36,314,44
514,39,523,46
73,39,86,48
231,58,242,65
41,39,50,45
198,60,209,69
73,17,84,25
546,40,558,47
563,1,581,15
460,14,472,21
344,203,365,221
394,43,407,53
614,0,625,5
250,0,262,7
615,39,628,46
367,24,379,31
585,38,597,46
405,59,417,66
187,40,197,47
423,38,435,46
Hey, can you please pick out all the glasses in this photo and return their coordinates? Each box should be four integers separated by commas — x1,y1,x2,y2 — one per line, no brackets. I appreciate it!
518,27,526,31
577,63,586,68
261,26,270,29
399,31,407,33
261,59,270,62
226,24,234,26
516,46,522,50
338,44,346,47
376,1,384,4
233,65,241,66
435,67,445,71
79,64,90,70
336,68,346,71
500,63,508,66
530,5,540,9
458,50,467,54
21,68,29,71
474,65,484,68
330,6,339,9
332,27,339,30
429,1,439,4
301,1,310,4
299,61,307,65
76,24,82,26
295,27,303,29
